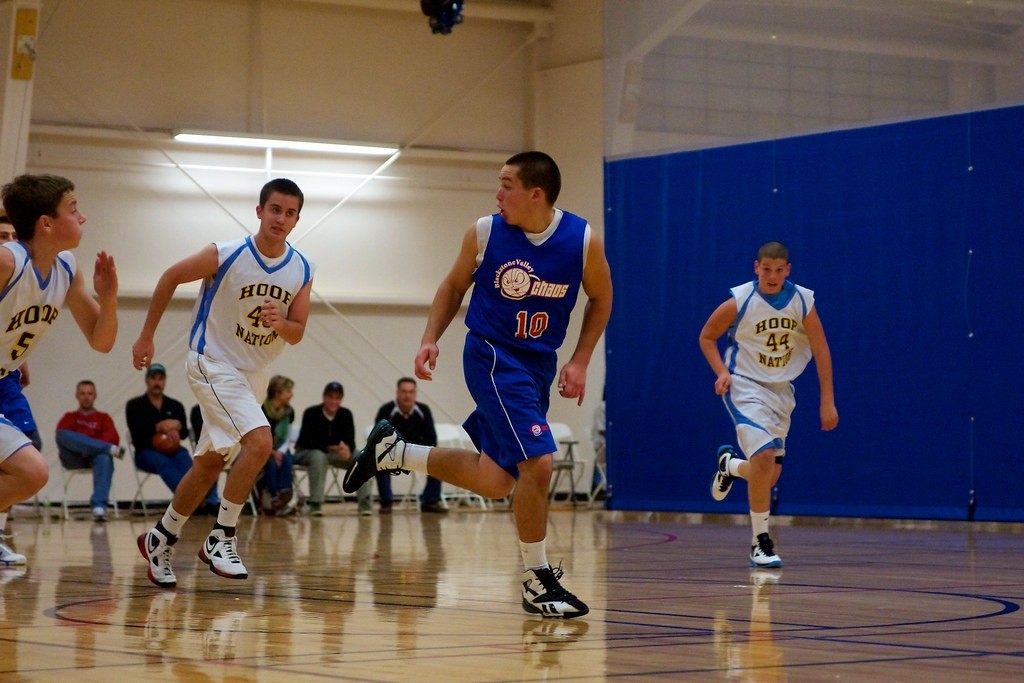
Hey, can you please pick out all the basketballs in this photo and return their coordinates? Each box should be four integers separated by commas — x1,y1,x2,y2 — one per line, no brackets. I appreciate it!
151,430,180,452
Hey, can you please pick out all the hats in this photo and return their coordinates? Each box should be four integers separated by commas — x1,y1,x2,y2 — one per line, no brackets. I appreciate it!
147,363,165,377
324,382,344,397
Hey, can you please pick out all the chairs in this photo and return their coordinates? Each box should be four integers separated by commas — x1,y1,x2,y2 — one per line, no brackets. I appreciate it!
188,429,259,518
124,431,171,521
289,420,607,516
55,451,120,520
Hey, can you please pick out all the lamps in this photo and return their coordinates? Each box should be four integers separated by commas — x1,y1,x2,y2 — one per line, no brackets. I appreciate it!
170,129,399,155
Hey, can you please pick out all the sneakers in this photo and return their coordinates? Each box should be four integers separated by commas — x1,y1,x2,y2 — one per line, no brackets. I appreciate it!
197,528,249,580
342,419,410,493
0,543,27,565
137,527,180,586
110,444,125,459
521,557,589,617
749,532,783,567
202,605,248,660
520,617,589,667
711,444,738,501
92,506,109,522
144,587,186,649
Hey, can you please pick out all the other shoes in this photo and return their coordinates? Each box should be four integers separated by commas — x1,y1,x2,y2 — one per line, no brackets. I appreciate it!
421,500,451,512
309,502,322,517
379,503,392,514
275,504,299,517
263,494,275,517
359,503,373,516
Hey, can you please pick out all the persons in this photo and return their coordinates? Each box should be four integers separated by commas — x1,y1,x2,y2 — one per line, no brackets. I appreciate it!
259,376,304,517
55,380,127,522
343,150,613,618
374,377,450,513
295,382,373,515
0,173,119,565
592,402,606,459
126,364,220,517
700,243,839,567
190,403,289,514
132,179,318,585
